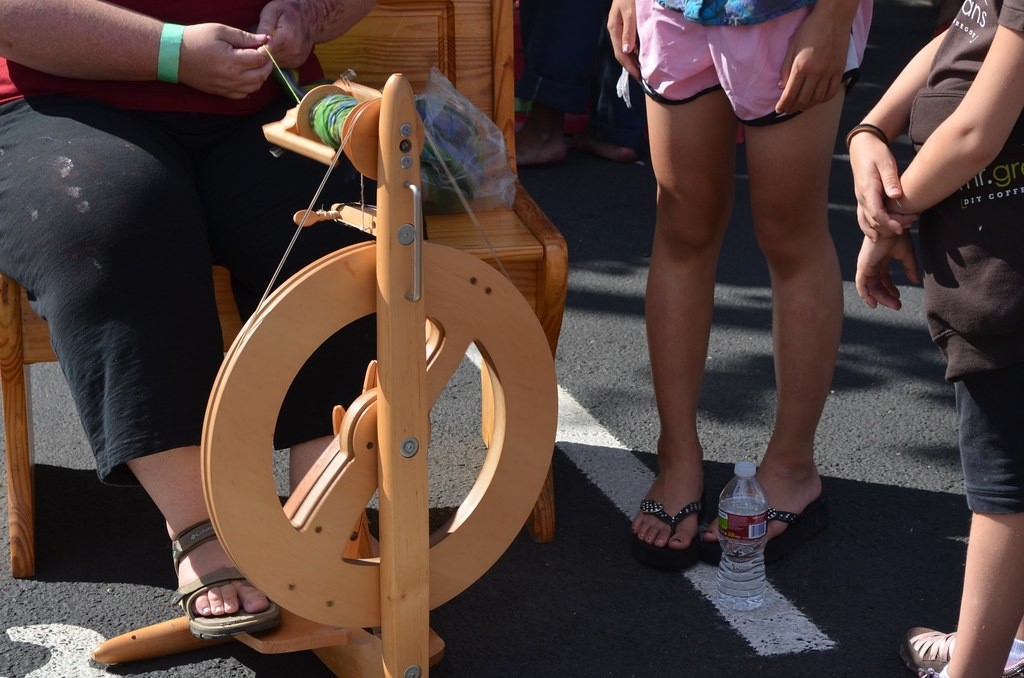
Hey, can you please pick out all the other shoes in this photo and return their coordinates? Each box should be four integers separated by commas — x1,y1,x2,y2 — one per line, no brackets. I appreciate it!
900,626,1024,678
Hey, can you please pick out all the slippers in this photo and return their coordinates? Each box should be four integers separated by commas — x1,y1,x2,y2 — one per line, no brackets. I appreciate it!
629,484,707,564
701,497,829,566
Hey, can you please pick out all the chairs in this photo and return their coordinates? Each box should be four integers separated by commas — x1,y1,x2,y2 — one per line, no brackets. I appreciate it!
0,0,572,580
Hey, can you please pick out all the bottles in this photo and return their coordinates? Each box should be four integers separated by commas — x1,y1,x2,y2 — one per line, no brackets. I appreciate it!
715,462,769,613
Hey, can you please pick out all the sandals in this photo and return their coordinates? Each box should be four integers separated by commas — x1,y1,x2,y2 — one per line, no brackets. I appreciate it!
171,518,281,640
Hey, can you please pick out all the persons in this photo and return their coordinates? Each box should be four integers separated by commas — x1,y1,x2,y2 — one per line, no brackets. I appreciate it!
845,0,1023,678
0,0,380,640
606,0,872,574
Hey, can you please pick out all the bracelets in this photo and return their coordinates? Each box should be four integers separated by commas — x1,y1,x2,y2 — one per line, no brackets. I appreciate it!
846,123,889,148
157,23,184,84
